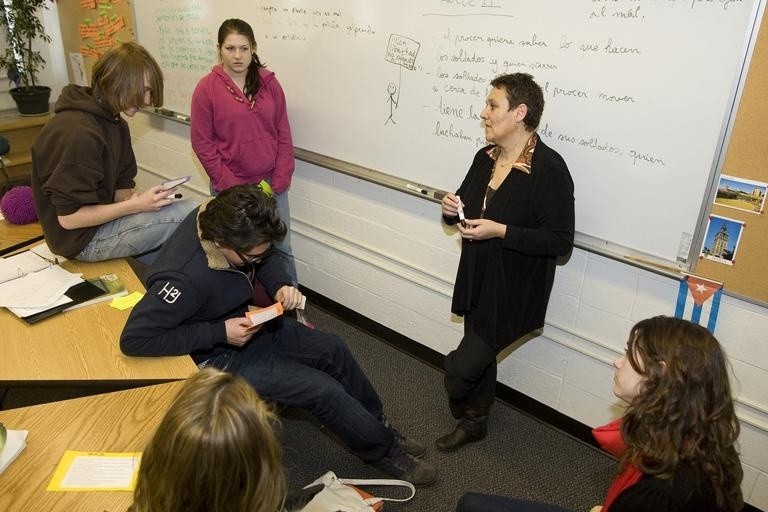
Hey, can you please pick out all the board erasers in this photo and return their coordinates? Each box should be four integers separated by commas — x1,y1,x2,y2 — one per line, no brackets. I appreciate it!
434,190,449,200
155,109,173,116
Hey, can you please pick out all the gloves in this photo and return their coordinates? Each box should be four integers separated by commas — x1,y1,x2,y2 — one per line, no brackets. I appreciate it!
285,483,324,511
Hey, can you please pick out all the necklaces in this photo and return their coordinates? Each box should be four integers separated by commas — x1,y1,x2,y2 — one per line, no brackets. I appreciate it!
499,151,517,168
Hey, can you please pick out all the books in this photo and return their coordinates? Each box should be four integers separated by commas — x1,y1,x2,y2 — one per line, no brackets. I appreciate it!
46,450,145,493
0,242,128,326
1,424,30,476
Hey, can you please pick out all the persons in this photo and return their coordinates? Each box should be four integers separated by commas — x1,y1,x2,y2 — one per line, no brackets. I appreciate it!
117,183,440,488
434,69,576,452
188,18,316,330
123,367,328,512
454,314,748,512
31,39,204,267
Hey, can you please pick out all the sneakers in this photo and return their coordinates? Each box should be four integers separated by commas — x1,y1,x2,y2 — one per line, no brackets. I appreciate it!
374,446,436,488
391,429,426,457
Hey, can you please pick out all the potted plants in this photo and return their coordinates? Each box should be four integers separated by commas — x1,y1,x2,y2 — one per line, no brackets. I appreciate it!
0,0,53,114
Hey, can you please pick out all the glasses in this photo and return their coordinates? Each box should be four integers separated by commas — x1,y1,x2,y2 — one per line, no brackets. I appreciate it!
234,247,275,266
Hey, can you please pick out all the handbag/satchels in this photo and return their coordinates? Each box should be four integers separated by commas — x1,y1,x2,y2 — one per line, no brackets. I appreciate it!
294,470,377,512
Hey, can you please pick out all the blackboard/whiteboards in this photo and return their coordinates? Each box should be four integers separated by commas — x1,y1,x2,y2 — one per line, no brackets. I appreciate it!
131,0,766,275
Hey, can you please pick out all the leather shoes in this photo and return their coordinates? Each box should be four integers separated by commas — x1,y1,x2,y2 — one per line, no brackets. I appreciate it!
445,395,474,418
434,411,488,451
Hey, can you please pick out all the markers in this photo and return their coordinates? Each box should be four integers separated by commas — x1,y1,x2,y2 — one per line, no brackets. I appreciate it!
406,183,428,195
177,115,190,121
456,196,466,228
165,194,182,199
